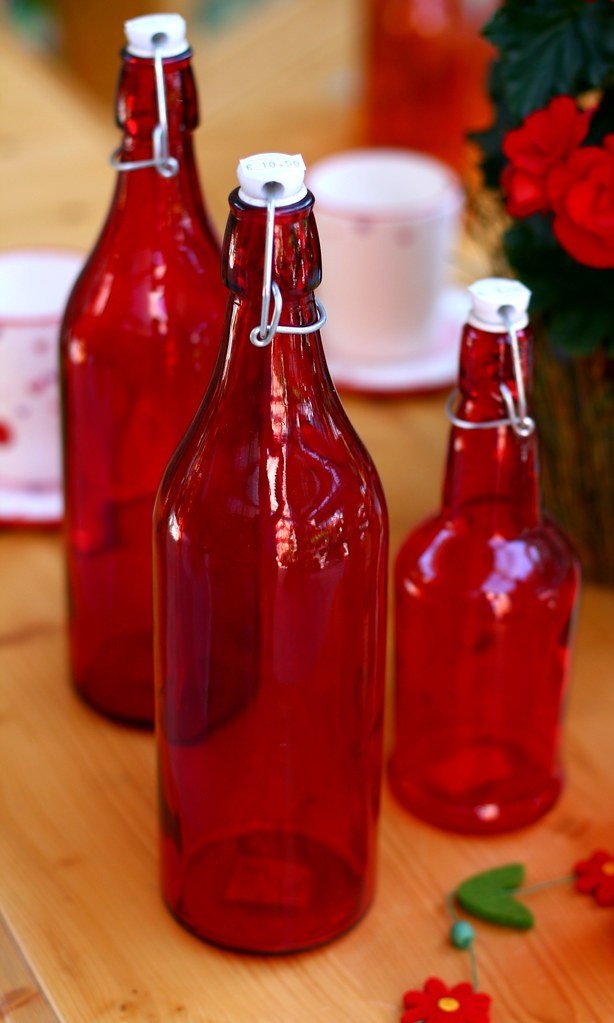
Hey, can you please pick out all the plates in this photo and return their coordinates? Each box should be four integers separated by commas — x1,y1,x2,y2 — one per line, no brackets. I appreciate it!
0,490,65,526
321,296,471,395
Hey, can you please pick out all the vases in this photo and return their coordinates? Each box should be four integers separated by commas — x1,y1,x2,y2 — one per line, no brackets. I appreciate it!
524,340,614,588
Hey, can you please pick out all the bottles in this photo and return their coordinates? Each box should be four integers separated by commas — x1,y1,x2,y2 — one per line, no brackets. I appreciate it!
58,40,270,731
365,0,509,202
392,320,584,834
153,186,392,959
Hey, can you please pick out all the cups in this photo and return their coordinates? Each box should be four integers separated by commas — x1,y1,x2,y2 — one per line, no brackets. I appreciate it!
0,253,87,495
308,155,464,366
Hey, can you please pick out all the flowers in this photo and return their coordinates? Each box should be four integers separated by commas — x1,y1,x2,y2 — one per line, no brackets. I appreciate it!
462,0,614,352
